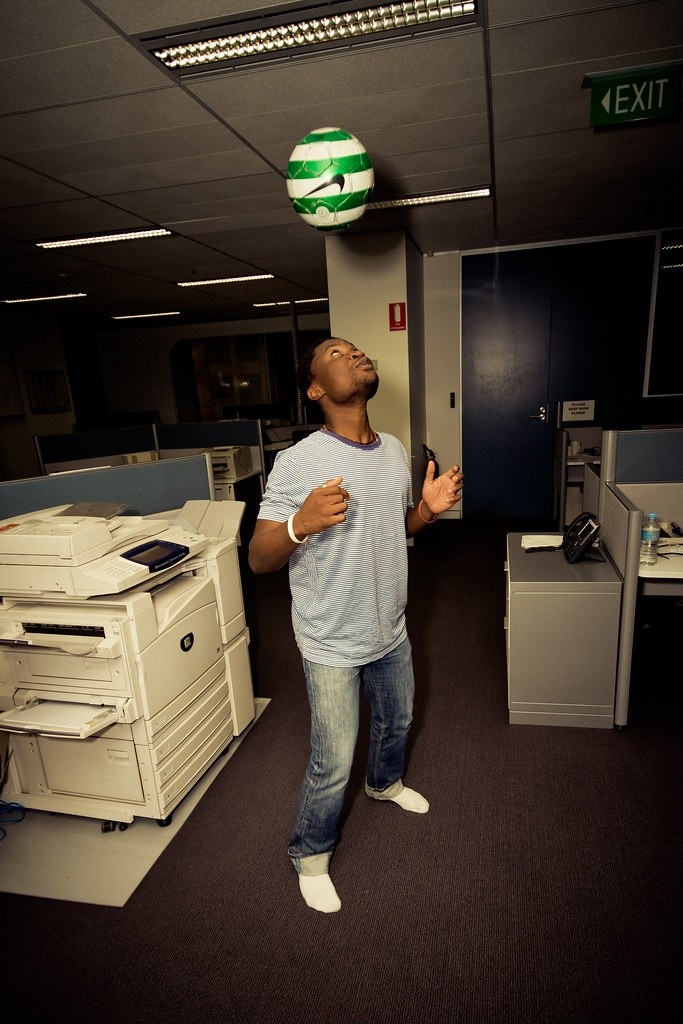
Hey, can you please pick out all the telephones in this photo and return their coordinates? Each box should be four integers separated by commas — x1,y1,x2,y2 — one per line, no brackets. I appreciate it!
563,511,606,565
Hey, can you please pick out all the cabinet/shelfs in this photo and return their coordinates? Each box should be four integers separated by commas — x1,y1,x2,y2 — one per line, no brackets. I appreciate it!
501,530,622,729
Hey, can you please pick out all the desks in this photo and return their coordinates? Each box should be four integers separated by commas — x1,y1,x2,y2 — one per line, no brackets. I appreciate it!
210,465,264,549
564,446,683,728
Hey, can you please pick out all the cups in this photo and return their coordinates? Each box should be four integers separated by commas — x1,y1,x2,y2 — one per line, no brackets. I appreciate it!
568,446,571,455
571,441,580,454
594,448,600,455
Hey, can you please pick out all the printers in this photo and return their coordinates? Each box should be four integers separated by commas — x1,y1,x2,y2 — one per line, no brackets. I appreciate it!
0,445,255,824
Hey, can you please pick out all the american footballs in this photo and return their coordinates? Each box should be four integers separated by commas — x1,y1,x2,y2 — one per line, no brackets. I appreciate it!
283,123,376,234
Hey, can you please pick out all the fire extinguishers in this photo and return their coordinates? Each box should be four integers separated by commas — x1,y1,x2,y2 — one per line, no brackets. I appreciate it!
422,443,439,479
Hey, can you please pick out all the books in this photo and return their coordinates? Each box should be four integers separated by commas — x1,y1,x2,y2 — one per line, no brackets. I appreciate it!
52,501,131,520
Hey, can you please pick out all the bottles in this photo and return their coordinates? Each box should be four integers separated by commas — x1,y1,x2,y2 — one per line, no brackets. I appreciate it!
639,512,661,565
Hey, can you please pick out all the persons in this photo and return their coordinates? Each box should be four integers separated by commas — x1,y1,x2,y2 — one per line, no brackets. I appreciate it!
248,336,463,914
